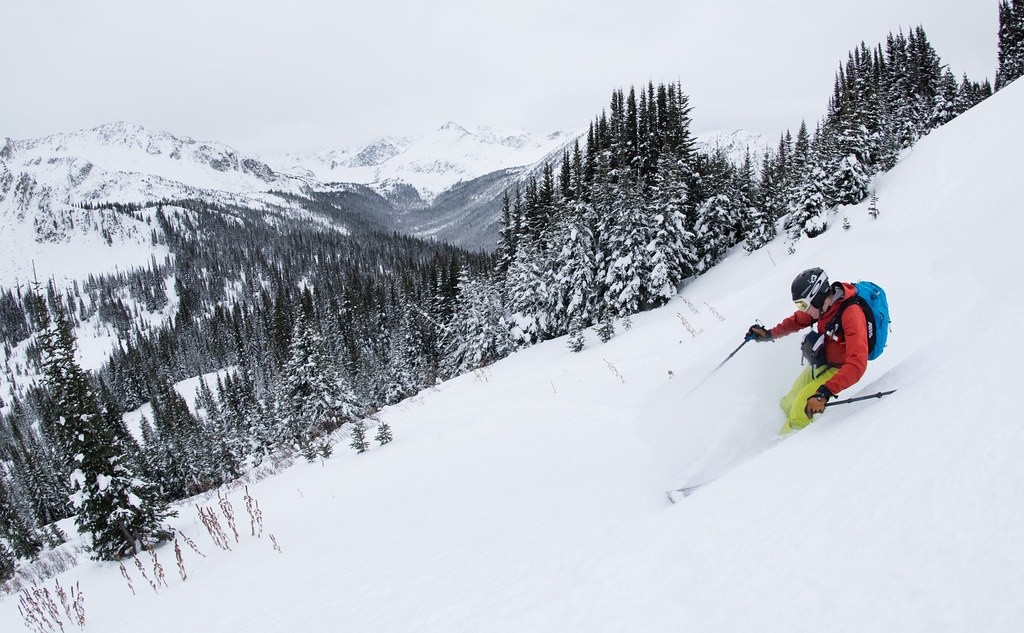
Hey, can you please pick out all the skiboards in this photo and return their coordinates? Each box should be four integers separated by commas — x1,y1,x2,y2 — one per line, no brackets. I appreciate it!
664,482,708,505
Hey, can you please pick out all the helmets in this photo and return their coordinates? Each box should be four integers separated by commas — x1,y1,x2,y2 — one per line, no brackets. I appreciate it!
791,267,831,309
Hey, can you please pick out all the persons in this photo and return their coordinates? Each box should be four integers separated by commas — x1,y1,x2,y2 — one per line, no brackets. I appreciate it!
745,267,876,435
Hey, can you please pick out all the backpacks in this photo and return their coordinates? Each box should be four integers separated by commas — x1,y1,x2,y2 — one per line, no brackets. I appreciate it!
834,281,891,360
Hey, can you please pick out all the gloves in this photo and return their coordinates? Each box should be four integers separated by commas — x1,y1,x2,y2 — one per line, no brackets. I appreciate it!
807,385,833,419
745,324,773,343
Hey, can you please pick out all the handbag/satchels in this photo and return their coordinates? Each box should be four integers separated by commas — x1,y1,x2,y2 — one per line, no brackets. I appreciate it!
800,330,828,369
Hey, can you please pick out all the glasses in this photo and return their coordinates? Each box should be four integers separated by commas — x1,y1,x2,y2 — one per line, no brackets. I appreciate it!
795,300,810,312
